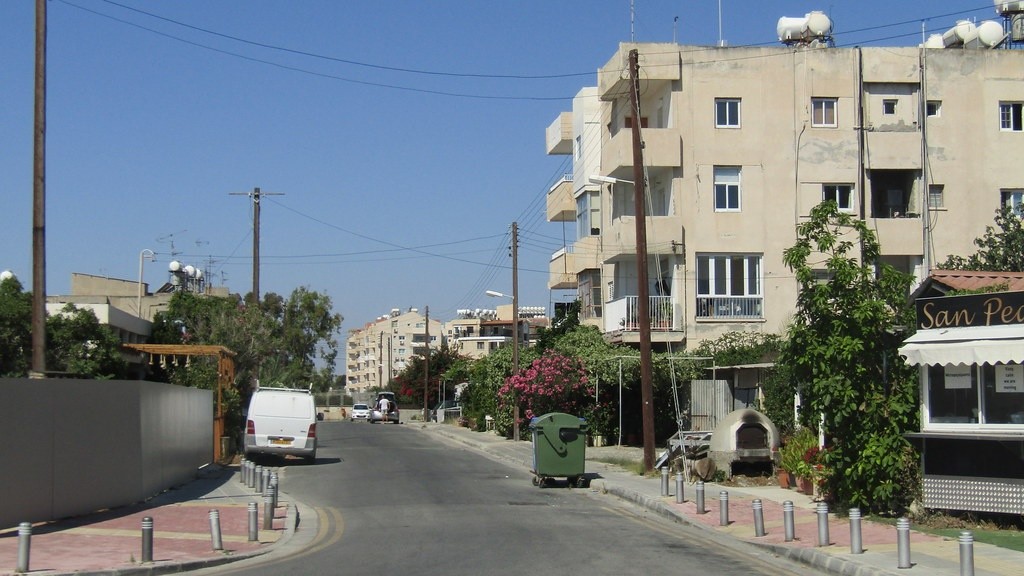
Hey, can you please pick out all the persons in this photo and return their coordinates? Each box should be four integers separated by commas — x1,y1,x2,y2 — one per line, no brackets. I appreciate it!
378,394,391,422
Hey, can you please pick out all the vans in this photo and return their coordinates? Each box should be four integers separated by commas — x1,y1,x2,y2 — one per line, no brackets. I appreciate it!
243,387,324,465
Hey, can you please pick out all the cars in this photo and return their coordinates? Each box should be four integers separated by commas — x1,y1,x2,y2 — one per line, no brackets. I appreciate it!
372,391,401,424
350,403,371,422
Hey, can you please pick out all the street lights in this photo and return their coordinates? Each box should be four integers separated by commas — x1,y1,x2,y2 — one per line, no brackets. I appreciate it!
136,248,157,315
588,173,658,475
485,290,521,442
